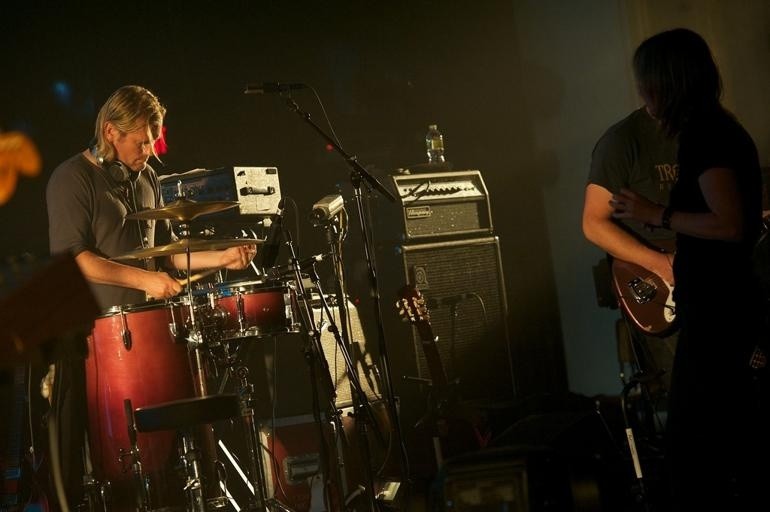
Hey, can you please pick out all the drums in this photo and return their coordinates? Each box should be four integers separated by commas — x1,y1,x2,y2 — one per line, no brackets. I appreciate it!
191,275,301,343
81,294,227,512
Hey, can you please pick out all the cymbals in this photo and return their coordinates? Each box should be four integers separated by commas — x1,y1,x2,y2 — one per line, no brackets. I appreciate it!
123,198,241,222
109,239,265,261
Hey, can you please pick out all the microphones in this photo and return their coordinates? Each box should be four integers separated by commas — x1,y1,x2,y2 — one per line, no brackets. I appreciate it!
244,81,306,97
262,253,331,284
261,199,287,268
427,293,472,309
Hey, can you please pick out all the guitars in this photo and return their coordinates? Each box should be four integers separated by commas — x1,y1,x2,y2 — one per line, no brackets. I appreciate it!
395,285,493,512
612,214,770,336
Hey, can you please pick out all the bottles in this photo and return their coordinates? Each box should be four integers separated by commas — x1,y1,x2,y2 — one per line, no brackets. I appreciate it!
424,124,446,163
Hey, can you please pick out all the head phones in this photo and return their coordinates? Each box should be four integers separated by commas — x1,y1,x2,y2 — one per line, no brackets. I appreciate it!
88,136,141,183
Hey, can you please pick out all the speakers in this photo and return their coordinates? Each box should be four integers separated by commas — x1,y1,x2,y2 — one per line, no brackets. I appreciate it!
374,234,526,474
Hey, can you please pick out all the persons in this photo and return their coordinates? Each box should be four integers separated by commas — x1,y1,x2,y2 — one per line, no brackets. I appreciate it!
46,85,257,314
582,27,763,432
